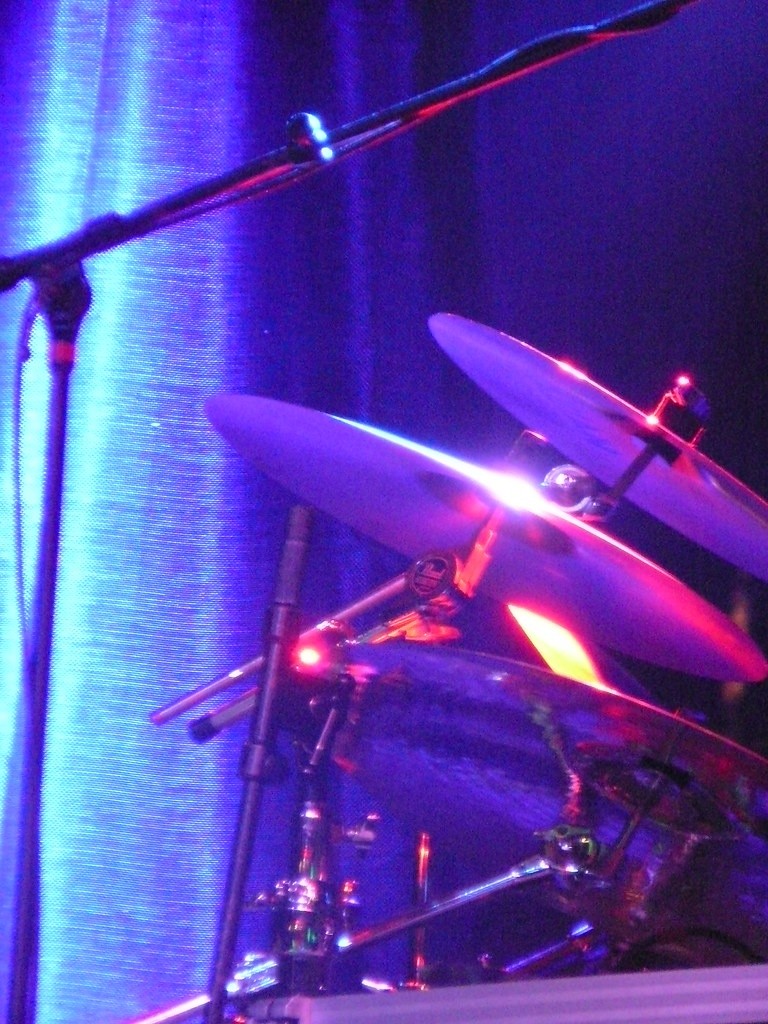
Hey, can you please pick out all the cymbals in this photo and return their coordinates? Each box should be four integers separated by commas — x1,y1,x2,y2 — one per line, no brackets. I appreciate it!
298,637,768,969
201,387,768,684
199,307,768,587
